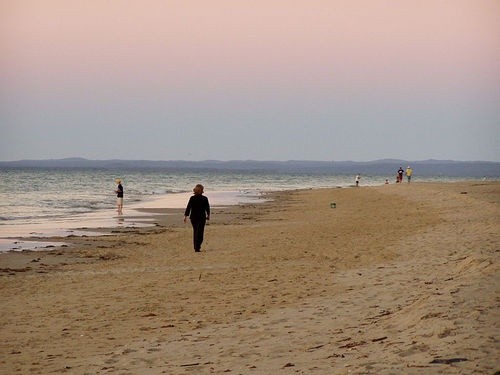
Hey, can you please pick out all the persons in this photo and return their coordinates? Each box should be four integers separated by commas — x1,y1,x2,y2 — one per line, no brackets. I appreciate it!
114,179,124,212
356,173,361,187
384,176,399,184
397,167,404,183
184,184,210,252
405,166,412,182
